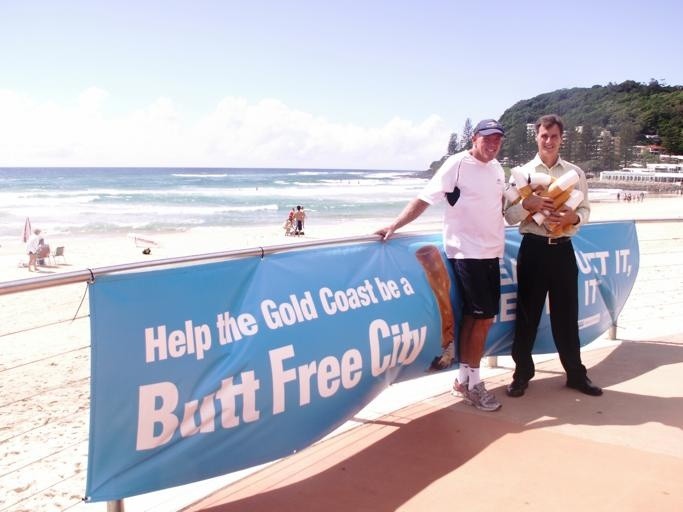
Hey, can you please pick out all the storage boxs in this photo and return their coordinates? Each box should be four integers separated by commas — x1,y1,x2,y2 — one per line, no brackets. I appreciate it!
36,259,45,265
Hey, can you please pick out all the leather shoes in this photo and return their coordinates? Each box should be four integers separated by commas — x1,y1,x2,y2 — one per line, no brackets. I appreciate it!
565,375,603,395
507,378,530,396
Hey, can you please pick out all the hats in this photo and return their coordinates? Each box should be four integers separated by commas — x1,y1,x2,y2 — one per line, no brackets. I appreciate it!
472,118,507,138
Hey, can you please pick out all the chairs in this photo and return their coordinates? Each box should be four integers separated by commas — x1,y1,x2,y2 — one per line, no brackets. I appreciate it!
28,248,50,267
49,245,67,266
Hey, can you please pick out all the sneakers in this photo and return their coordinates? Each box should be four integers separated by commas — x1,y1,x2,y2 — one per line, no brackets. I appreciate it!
451,378,502,411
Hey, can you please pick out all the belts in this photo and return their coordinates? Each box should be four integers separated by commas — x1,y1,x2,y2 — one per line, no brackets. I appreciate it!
523,233,569,245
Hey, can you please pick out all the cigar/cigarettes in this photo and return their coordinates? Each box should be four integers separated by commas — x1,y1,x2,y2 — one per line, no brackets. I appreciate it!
508,165,585,238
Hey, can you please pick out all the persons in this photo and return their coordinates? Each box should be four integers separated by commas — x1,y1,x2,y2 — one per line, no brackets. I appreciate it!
25,228,40,272
35,238,50,258
372,118,509,413
614,191,645,204
281,204,305,237
504,112,607,398
